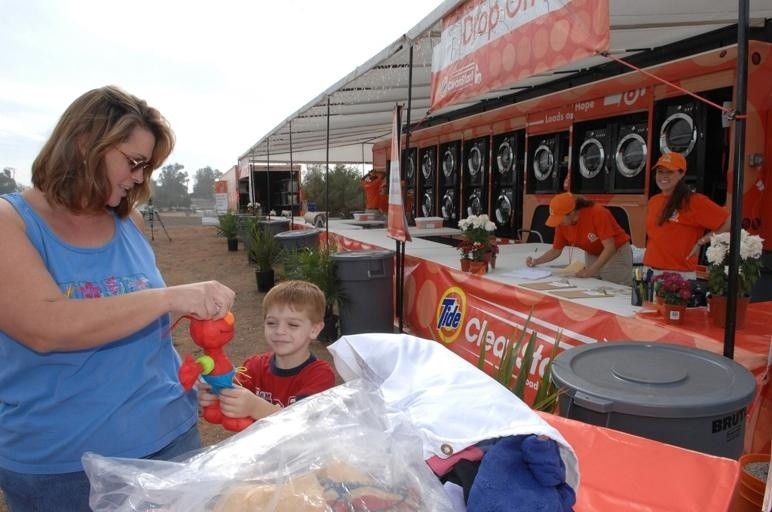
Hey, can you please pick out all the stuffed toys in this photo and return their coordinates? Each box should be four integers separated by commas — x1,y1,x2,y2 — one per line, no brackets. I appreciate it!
163,307,254,432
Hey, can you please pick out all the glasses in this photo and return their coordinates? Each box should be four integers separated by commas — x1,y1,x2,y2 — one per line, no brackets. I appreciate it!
112,145,151,173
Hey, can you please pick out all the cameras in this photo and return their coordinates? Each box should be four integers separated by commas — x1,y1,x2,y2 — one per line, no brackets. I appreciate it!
148,196,153,205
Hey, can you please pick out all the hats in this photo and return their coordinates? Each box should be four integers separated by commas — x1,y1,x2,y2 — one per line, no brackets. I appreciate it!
545,192,576,227
651,151,687,176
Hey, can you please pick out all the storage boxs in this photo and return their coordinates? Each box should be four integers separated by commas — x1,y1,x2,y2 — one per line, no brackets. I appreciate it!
353,213,368,220
414,216,444,228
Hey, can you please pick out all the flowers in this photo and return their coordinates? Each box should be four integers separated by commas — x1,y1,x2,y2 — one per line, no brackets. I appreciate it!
705,229,765,296
455,238,473,259
650,272,692,305
458,214,497,239
473,239,499,260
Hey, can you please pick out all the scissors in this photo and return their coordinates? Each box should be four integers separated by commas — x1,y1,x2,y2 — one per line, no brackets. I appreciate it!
646,269,653,281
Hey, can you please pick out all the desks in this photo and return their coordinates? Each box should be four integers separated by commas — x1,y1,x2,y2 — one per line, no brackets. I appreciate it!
530,410,741,511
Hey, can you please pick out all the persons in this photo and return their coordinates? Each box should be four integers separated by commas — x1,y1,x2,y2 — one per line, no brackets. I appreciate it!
526,192,633,286
0,85,237,512
379,184,388,216
643,153,731,304
400,181,413,226
196,280,336,439
359,169,386,219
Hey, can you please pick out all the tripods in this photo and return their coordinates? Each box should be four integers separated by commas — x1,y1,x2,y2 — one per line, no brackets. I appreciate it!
142,205,172,243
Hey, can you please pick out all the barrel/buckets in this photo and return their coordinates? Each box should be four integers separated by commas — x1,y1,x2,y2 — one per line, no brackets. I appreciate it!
259,219,289,235
276,229,320,272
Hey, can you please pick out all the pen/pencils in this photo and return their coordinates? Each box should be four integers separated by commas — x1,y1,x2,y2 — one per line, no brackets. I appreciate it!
632,267,646,306
602,288,606,295
530,248,537,267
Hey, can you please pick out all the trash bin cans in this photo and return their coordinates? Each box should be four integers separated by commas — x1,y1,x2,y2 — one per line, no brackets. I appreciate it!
274,229,320,259
257,220,290,235
218,214,232,224
232,214,253,217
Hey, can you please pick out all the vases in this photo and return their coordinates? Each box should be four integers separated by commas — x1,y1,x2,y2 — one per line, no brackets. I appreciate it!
664,302,687,324
460,259,472,272
485,261,488,271
470,261,485,274
711,291,752,328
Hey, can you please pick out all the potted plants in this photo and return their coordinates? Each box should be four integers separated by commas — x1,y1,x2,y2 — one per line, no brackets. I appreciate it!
284,245,354,344
217,215,242,251
246,223,287,295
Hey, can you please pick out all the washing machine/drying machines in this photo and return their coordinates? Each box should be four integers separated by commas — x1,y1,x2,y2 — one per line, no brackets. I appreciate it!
400,147,417,187
569,116,612,196
437,186,459,228
489,187,523,240
461,187,488,219
437,139,461,186
489,128,526,186
525,131,570,198
419,188,436,217
648,86,734,207
611,112,646,195
419,144,437,188
462,135,491,187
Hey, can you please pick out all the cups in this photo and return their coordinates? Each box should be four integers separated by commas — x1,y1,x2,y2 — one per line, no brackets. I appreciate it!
644,282,653,302
631,278,646,307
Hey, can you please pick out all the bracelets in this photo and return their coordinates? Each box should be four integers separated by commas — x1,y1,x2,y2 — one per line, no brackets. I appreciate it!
696,238,705,248
708,231,716,237
